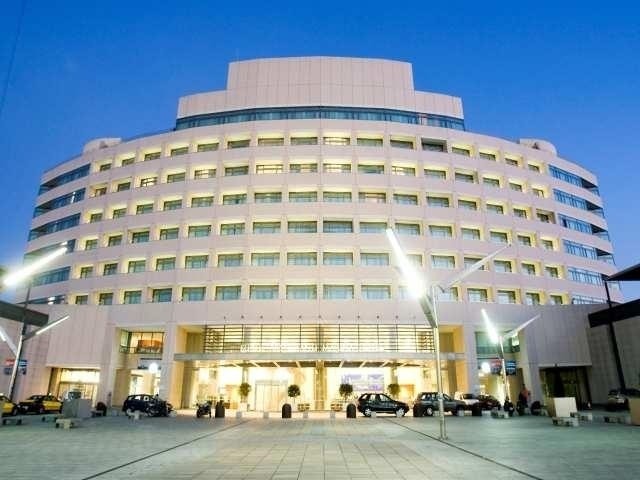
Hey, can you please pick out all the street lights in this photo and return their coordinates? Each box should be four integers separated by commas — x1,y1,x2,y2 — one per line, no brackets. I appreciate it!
0,247,69,402
385,228,512,439
481,308,540,412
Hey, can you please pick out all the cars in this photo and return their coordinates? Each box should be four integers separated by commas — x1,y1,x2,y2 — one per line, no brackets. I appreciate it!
608,388,640,410
358,394,409,417
415,390,500,416
0,393,61,417
122,394,172,416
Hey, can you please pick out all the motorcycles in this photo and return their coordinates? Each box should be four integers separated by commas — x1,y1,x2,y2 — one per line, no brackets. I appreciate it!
196,401,212,417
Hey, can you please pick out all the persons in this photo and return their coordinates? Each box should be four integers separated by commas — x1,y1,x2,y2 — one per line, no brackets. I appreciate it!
515,393,528,416
504,396,514,416
519,384,530,402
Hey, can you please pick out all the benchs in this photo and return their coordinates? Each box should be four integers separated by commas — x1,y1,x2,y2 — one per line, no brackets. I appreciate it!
482,408,633,428
3,412,150,430
